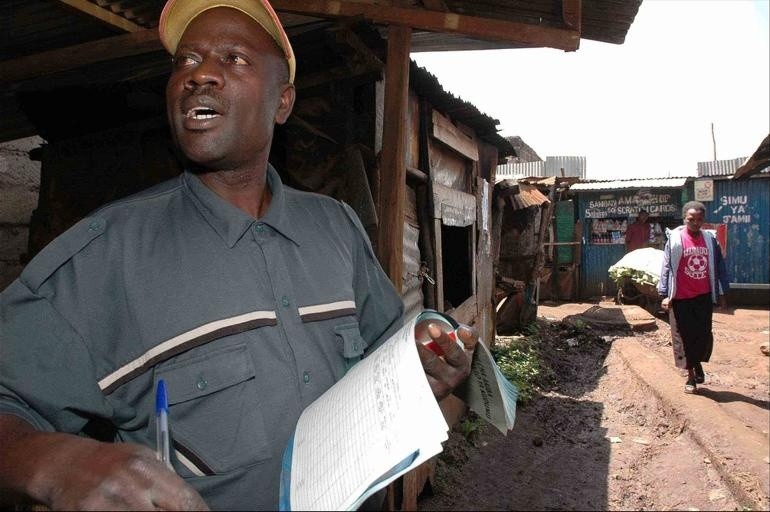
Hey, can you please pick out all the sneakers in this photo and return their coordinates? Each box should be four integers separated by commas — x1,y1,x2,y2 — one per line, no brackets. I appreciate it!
694,364,704,382
685,380,696,394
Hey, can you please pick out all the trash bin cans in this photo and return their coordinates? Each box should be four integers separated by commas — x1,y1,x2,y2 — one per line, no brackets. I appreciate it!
556,263,574,300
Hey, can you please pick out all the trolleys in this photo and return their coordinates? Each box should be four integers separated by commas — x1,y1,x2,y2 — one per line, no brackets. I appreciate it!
617,278,668,314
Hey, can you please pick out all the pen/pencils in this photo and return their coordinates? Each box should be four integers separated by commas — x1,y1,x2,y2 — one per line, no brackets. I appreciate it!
154,380,170,464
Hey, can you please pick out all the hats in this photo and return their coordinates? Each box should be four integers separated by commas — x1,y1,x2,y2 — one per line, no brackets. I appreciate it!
158,1,297,83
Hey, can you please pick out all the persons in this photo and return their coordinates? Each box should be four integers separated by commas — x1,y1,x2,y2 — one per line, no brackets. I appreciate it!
659,201,730,394
625,210,650,253
0,0,477,512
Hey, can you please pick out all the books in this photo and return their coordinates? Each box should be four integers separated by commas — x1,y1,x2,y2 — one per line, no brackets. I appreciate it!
279,308,520,512
